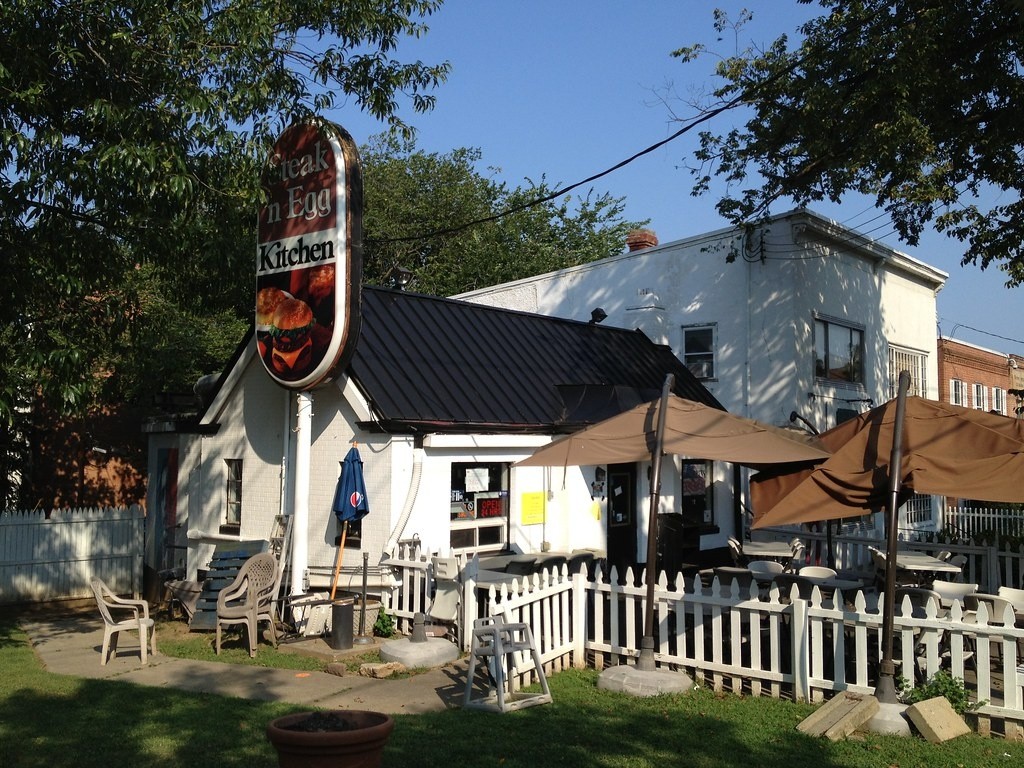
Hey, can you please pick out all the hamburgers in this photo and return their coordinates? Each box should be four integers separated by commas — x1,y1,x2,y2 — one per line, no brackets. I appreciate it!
253,286,319,374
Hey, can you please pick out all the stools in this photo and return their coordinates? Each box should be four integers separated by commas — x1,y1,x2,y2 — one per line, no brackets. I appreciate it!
464,623,552,713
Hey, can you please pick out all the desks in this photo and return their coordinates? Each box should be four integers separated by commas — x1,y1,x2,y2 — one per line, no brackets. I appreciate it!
940,597,1024,626
699,567,864,609
877,550,962,590
742,542,793,567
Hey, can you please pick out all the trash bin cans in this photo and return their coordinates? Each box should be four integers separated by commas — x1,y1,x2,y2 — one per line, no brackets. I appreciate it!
332,602,356,650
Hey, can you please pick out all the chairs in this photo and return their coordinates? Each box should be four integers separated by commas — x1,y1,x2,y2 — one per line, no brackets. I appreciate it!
89,575,156,665
216,554,282,657
713,536,1024,683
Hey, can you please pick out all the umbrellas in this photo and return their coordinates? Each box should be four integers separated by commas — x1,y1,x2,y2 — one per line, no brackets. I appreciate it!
327,441,369,601
510,392,832,669
749,394,1023,703
